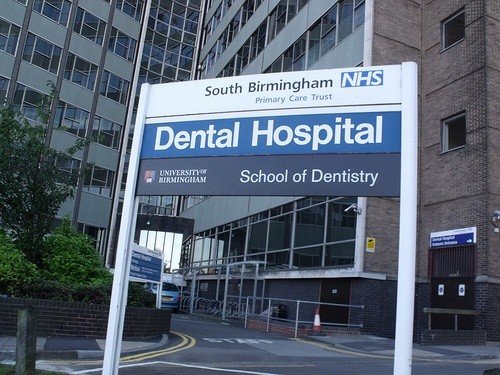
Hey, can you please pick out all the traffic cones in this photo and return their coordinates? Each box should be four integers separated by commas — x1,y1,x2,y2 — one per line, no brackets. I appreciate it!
307,305,328,337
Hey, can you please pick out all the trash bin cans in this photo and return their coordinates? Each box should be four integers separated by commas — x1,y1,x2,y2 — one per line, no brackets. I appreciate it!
272,304,287,321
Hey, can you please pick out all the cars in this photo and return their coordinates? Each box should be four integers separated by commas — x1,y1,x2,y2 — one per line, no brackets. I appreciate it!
146,281,182,314
